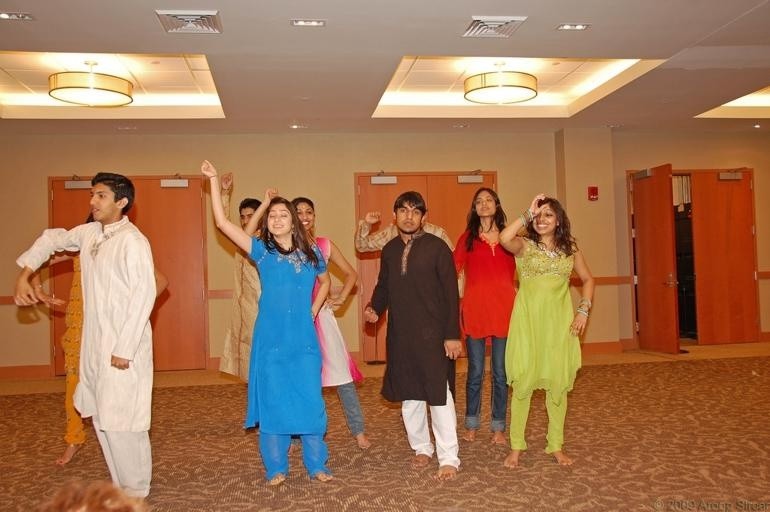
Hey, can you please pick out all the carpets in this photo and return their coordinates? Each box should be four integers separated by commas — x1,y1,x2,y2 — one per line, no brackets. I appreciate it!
0,355,770,512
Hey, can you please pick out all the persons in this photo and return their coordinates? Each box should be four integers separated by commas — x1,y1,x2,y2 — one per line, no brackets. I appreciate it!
14,170,158,502
201,159,334,487
220,174,267,383
452,186,522,447
354,210,464,403
365,191,464,481
497,192,596,471
291,197,371,449
30,214,171,467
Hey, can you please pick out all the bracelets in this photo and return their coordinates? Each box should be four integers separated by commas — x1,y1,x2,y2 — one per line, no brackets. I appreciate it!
210,174,218,178
520,208,534,228
576,308,589,318
32,285,43,289
577,297,592,309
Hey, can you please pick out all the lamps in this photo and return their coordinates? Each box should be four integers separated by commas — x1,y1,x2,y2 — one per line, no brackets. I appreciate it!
463,64,538,104
48,60,134,106
